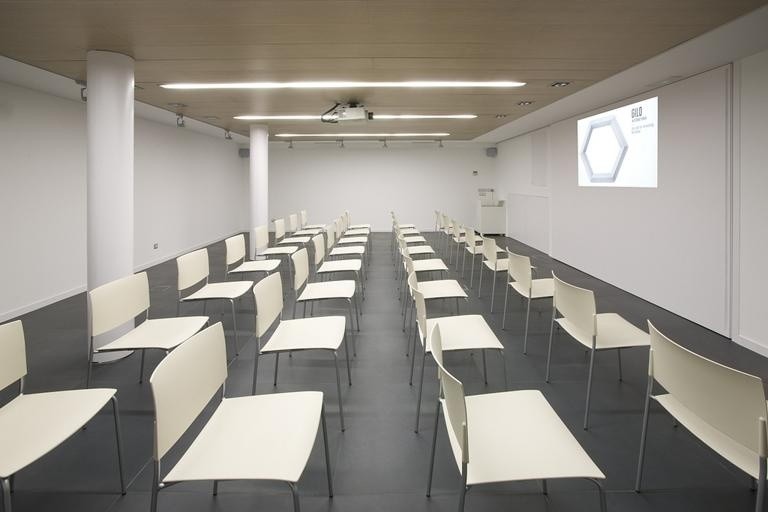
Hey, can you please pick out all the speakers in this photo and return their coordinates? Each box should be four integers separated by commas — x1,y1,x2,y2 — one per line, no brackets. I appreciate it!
239,148,249,157
486,147,497,156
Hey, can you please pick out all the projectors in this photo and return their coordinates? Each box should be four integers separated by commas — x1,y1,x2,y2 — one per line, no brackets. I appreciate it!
336,107,375,121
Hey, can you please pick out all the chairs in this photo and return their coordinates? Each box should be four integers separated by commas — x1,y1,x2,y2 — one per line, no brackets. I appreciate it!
144,318,334,512
0,319,129,511
250,271,353,436
288,247,361,357
386,204,768,511
221,209,373,333
80,268,210,430
175,248,256,358
